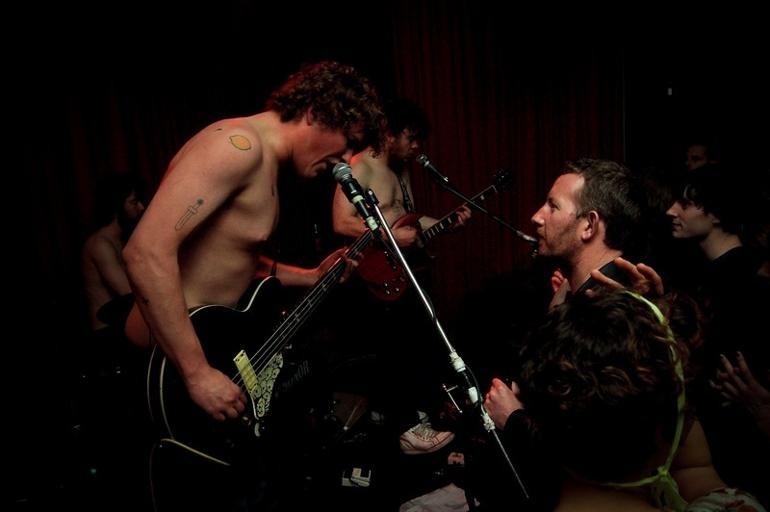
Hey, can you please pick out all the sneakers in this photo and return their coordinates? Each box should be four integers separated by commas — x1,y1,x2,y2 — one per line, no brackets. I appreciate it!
399,411,456,454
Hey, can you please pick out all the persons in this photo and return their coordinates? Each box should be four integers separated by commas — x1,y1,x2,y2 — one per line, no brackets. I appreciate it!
483,160,769,512
81,59,472,511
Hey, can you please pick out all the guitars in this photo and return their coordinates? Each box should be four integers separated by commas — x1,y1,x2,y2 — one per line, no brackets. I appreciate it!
148,228,381,462
357,173,503,300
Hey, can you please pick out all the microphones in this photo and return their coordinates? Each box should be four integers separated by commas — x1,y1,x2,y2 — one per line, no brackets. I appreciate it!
331,159,382,240
414,149,451,191
